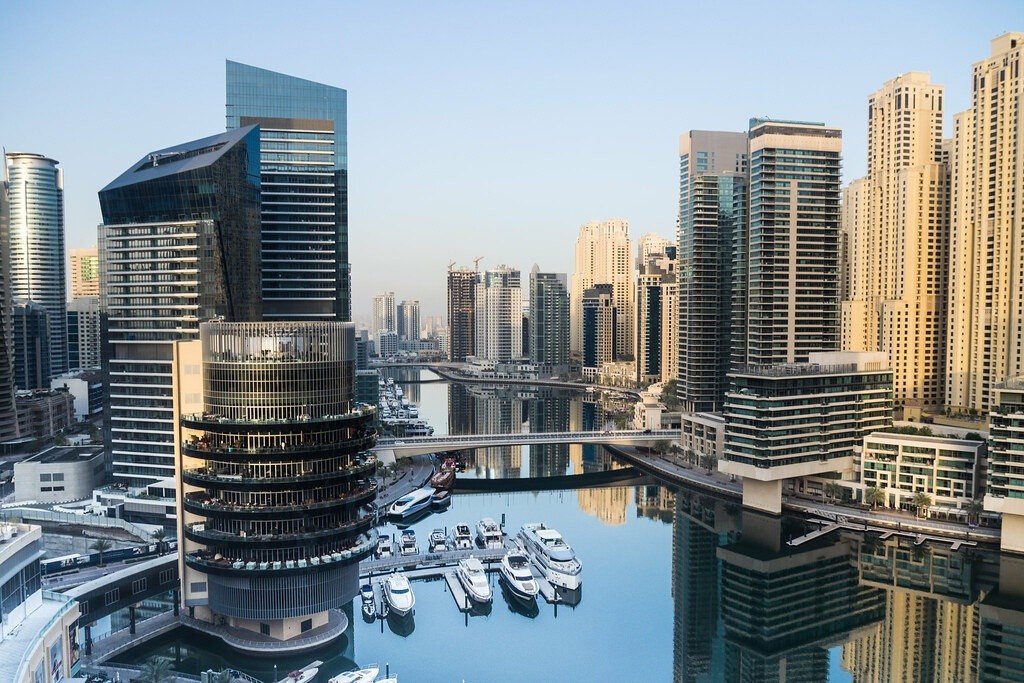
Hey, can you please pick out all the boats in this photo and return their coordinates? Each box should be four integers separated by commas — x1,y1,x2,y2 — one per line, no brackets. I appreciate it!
375,535,395,560
451,524,476,550
498,550,540,601
518,523,584,591
386,488,437,518
381,573,416,617
429,528,449,551
551,582,581,607
398,529,419,555
359,585,376,617
457,556,492,604
431,490,451,506
387,609,416,639
467,593,492,619
279,663,398,683
477,517,506,550
363,612,376,625
465,384,486,396
498,576,539,619
428,450,466,489
386,377,434,435
576,385,631,399
